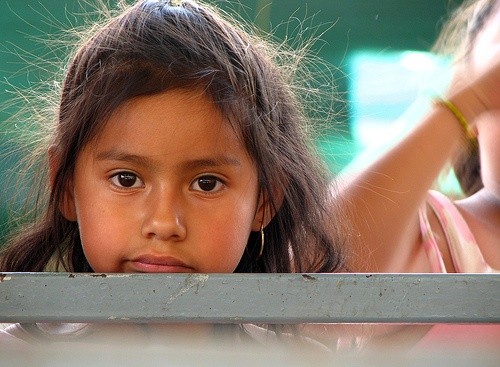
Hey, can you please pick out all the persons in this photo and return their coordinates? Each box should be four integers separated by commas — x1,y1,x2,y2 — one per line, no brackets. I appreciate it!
266,1,499,367
1,1,345,367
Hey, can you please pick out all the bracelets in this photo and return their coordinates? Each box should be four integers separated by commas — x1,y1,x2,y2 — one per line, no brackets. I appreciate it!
430,95,478,140
